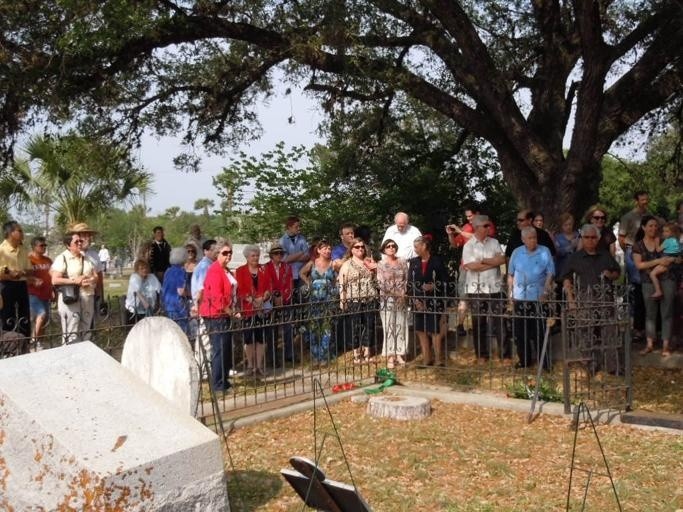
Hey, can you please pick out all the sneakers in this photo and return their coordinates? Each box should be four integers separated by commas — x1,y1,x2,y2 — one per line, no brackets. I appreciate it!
245,368,252,376
458,326,466,335
28,338,35,353
257,367,268,375
35,340,43,351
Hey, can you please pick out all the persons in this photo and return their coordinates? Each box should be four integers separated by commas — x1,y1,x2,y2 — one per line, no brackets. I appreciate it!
298,204,512,370
0,221,111,345
124,217,308,397
506,191,682,376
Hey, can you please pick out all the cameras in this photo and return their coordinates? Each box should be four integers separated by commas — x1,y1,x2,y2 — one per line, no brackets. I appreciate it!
450,227,456,233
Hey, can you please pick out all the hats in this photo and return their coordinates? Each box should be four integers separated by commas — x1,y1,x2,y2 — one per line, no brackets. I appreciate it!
68,223,95,233
268,243,286,253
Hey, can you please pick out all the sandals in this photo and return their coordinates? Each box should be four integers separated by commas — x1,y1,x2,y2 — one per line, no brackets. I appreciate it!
632,332,675,358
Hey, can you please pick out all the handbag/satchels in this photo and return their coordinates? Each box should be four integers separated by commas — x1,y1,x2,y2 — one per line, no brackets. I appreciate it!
60,275,78,304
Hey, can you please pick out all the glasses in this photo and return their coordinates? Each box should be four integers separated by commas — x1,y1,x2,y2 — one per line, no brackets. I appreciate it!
38,243,47,247
74,239,83,243
480,215,606,239
272,251,284,255
187,249,194,253
222,250,231,256
386,245,395,248
352,246,365,249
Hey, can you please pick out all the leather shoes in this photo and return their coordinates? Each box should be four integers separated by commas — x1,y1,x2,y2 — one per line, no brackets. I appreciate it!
588,361,598,373
272,359,280,368
284,357,300,363
308,353,555,374
